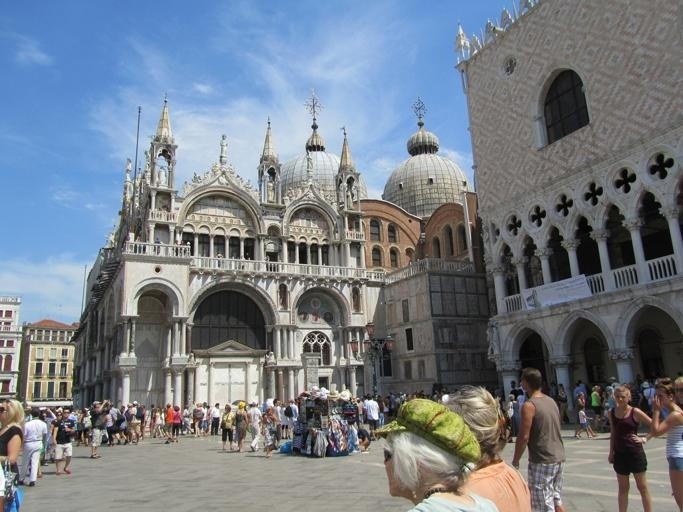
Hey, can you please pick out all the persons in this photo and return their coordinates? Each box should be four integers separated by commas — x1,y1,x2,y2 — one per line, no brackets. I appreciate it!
650,379,683,508
18,399,298,486
494,381,527,437
549,376,683,438
373,398,499,512
0,398,24,512
606,386,655,512
346,389,450,449
444,385,532,512
511,369,565,512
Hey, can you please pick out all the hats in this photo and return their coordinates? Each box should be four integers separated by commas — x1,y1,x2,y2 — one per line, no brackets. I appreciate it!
93,401,102,405
239,402,245,408
375,399,482,463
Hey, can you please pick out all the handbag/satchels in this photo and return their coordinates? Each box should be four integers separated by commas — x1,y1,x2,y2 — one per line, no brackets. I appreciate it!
284,406,293,417
3,470,17,501
221,421,226,430
136,407,144,418
116,414,125,426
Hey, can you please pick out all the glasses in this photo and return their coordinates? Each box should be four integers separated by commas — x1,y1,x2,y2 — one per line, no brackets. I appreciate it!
0,407,8,414
384,450,394,464
